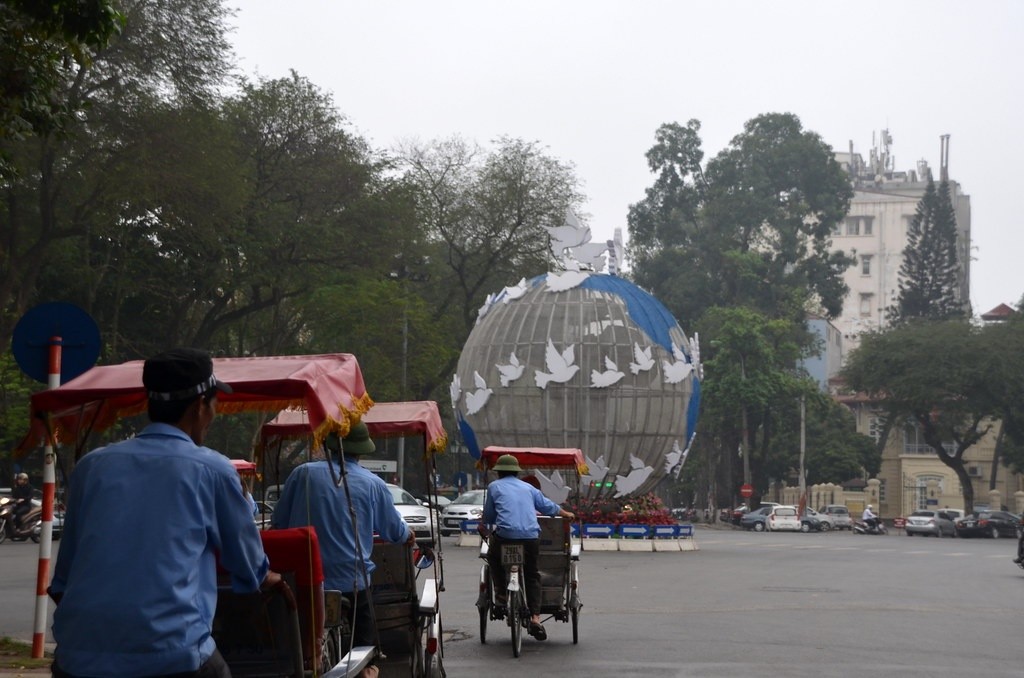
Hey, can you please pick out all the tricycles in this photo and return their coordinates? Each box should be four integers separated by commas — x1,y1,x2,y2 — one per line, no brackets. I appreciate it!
254,398,451,678
475,445,584,658
8,352,387,678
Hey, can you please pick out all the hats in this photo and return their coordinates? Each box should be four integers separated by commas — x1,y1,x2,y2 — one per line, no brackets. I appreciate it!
326,420,376,454
492,454,523,471
142,346,233,401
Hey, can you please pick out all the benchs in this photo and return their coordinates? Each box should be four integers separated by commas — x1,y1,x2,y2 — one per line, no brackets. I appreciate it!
212,526,377,678
476,515,581,605
331,531,441,665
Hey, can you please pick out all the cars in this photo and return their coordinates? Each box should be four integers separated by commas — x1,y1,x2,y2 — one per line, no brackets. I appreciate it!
719,501,855,533
373,484,437,546
954,510,1022,539
0,488,67,538
439,489,490,537
936,508,965,524
250,484,286,531
904,508,960,538
415,494,456,513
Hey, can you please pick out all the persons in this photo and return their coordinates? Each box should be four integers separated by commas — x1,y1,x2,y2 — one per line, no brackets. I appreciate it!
10,473,34,532
478,454,576,641
47,347,282,678
1013,510,1024,563
248,492,259,517
269,419,415,678
420,549,433,567
862,504,879,531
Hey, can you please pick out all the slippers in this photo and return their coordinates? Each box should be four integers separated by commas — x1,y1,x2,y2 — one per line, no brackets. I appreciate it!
494,607,507,616
531,622,547,641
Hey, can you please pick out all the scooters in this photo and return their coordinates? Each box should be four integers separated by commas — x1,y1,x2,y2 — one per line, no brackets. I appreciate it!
0,496,46,545
853,518,889,536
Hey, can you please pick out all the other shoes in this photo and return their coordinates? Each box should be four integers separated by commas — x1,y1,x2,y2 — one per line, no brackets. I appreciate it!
359,665,379,678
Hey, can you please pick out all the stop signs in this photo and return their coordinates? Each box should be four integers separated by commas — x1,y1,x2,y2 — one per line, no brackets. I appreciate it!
740,484,753,498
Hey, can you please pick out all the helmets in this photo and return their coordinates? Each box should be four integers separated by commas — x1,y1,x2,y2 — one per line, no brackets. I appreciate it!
17,473,28,486
